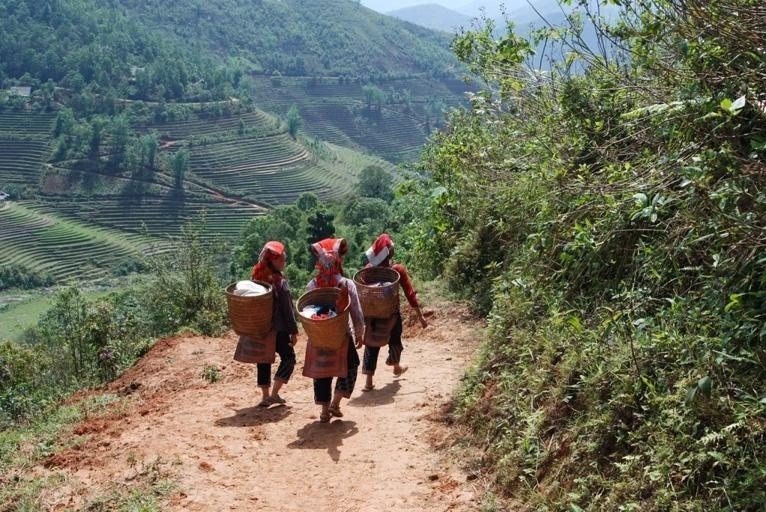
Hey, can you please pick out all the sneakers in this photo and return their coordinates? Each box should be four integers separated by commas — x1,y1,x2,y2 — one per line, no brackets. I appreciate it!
392,365,409,378
320,402,343,423
364,381,376,392
259,394,286,407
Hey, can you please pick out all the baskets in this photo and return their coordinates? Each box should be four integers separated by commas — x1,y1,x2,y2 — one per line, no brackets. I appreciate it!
295,286,352,350
352,266,401,319
224,279,275,341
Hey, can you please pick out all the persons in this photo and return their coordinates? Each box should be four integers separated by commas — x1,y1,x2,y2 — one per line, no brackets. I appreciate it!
360,233,429,392
230,240,299,409
301,237,366,424
